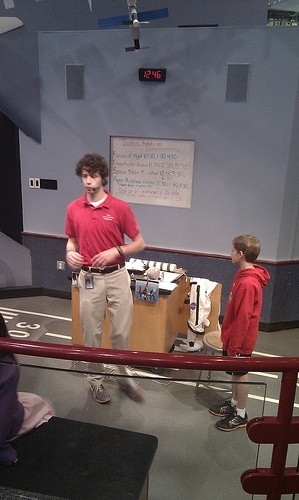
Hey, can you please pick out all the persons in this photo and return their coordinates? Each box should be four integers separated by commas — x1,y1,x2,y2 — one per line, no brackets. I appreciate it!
64,153,148,405
208,233,272,432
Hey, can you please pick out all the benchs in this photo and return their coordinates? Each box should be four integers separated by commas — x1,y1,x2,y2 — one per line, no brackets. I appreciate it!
0,415,159,500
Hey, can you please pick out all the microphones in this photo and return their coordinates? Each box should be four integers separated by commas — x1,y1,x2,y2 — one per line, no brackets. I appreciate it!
92,183,103,192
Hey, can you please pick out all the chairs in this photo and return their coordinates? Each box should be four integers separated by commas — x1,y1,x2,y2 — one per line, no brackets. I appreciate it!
188,277,221,350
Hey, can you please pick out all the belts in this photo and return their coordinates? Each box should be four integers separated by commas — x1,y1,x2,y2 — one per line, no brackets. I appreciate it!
81,261,125,274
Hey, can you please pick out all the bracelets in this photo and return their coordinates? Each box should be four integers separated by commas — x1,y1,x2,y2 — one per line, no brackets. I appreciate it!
65,249,74,255
116,244,128,259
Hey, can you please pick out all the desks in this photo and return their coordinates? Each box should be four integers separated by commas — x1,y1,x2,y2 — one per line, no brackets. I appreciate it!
70,264,189,374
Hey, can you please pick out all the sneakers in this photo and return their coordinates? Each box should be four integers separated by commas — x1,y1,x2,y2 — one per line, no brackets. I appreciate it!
88,383,111,403
120,380,147,402
209,401,249,432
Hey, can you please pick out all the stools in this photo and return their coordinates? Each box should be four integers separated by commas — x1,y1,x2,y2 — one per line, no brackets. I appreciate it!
193,331,234,396
